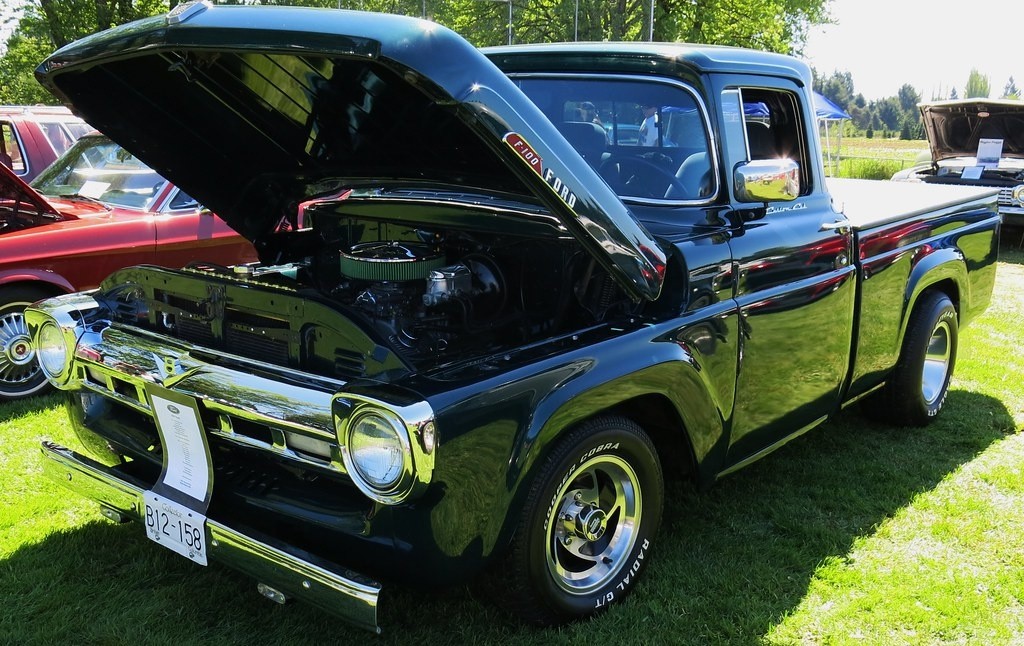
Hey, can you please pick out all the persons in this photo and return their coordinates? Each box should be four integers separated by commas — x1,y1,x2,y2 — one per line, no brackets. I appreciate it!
577,102,600,124
636,106,658,146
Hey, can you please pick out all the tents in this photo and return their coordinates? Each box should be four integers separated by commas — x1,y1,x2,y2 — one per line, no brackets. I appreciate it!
661,91,852,177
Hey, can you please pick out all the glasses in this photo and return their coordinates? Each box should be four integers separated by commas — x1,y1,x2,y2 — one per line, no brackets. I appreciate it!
640,103,656,110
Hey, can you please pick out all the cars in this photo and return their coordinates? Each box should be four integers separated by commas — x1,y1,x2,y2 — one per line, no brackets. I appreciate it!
0,104,355,406
890,96,1024,228
29,0,1007,637
599,122,679,148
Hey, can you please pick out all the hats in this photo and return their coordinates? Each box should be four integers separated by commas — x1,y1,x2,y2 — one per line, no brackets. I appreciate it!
581,102,596,111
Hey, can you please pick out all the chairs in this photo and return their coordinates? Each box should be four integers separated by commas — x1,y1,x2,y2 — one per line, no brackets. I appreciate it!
744,122,781,161
